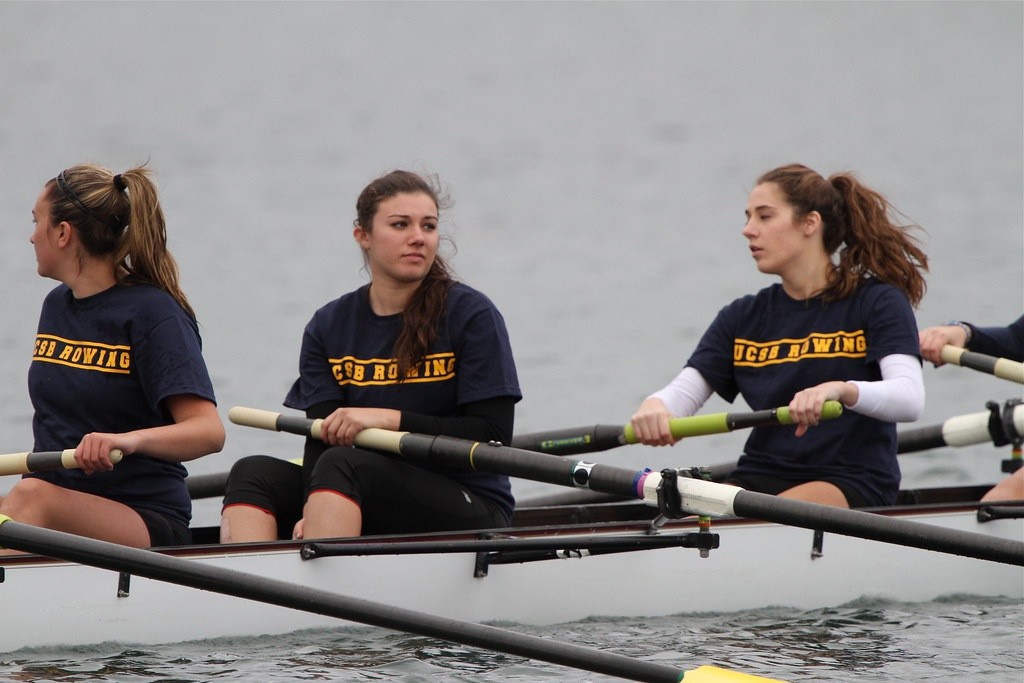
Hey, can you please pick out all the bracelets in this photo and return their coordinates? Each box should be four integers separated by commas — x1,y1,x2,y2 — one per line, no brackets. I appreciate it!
948,321,970,343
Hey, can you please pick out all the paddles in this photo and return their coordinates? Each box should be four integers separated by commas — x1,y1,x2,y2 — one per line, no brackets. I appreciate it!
0,513,793,683
0,448,126,476
227,405,1024,565
940,342,1024,383
513,399,1024,509
179,399,846,499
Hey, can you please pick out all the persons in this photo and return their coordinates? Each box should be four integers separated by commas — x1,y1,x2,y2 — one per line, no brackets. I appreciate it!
219,171,524,544
0,165,226,556
917,314,1024,502
631,164,931,509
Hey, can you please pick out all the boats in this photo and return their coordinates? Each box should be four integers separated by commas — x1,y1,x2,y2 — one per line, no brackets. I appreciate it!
0,480,1024,657
